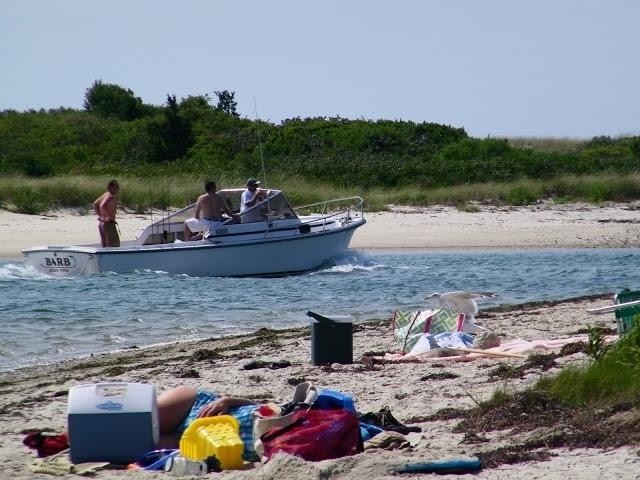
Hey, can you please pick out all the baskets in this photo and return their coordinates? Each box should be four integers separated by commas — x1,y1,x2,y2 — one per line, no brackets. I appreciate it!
179,415,244,469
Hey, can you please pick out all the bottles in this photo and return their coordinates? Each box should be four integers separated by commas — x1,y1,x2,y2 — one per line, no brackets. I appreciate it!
165,457,207,478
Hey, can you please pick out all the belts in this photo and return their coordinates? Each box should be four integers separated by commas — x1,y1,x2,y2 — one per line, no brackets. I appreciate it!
205,218,219,221
98,218,117,224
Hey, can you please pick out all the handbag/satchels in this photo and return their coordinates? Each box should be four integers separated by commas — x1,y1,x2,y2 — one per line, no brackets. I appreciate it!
394,308,466,356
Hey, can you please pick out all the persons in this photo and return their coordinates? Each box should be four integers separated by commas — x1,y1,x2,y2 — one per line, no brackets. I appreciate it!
93,180,120,247
240,178,267,224
184,181,240,241
157,385,261,462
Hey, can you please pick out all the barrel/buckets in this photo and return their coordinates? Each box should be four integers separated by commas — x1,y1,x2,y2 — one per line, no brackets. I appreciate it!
613,292,638,335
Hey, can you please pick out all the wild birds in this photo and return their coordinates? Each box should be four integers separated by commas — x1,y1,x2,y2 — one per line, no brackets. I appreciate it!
424,291,500,321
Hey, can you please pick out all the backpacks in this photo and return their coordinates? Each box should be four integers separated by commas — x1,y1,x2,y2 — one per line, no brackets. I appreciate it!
252,408,364,462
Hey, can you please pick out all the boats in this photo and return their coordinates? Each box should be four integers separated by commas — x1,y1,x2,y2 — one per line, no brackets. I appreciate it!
19,98,371,279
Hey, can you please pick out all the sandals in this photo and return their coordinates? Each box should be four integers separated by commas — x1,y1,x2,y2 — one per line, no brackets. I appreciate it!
138,448,181,471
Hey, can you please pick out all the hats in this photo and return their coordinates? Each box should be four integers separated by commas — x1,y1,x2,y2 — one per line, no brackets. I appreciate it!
247,178,261,187
